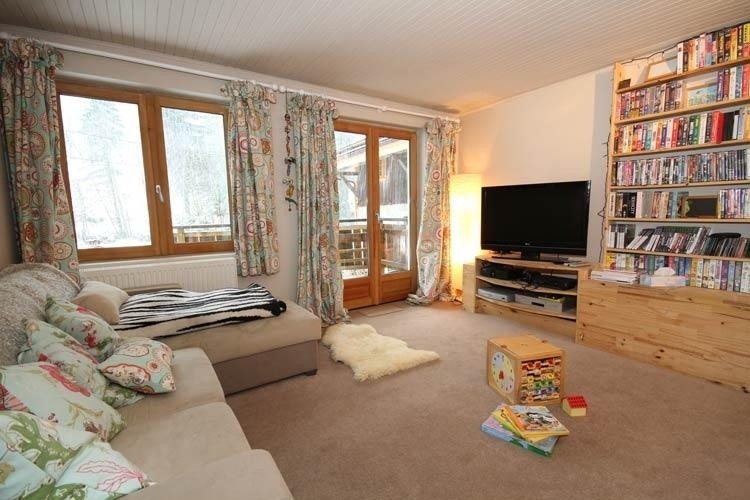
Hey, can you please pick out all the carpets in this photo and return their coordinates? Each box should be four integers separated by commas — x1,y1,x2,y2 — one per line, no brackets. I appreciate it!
323,316,438,384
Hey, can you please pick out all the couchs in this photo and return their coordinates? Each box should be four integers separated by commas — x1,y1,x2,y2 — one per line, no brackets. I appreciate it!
1,257,322,500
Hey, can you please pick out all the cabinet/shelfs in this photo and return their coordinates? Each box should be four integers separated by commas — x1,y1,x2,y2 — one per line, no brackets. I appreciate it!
474,254,592,338
576,25,750,396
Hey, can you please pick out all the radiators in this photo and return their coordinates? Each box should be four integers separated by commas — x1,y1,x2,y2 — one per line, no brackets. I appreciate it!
79,254,238,294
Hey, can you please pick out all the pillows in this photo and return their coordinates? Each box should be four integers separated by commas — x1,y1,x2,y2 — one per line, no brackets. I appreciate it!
1,277,177,500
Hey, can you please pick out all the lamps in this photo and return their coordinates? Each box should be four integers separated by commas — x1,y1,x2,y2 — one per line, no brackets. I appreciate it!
449,173,485,303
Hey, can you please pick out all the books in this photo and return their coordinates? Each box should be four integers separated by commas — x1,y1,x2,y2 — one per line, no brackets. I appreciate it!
591,223,750,294
608,191,689,219
482,402,570,457
718,187,750,219
677,21,750,74
611,64,750,186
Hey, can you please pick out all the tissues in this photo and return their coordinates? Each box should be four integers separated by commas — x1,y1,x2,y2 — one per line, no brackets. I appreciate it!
640,267,687,288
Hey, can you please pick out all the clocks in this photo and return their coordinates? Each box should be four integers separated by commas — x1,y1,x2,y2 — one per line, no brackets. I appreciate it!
486,344,516,398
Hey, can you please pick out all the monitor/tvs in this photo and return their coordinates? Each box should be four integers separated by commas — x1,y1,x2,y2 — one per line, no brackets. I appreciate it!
481,180,591,262
